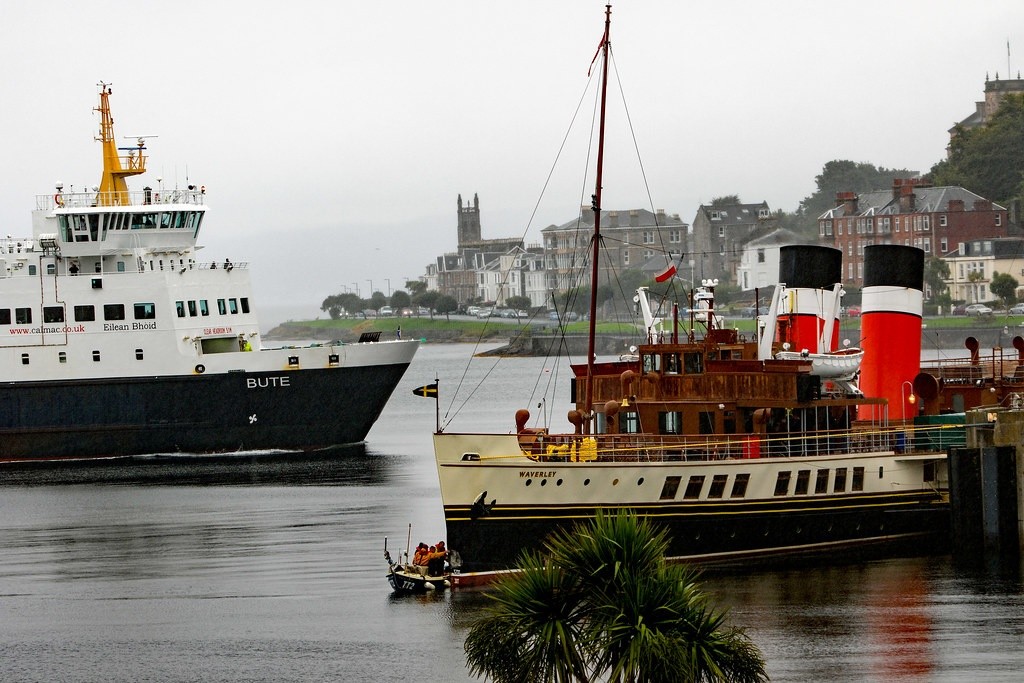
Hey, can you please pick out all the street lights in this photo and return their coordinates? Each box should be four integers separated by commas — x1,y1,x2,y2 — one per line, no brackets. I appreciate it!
367,279,373,296
384,278,390,296
340,283,361,296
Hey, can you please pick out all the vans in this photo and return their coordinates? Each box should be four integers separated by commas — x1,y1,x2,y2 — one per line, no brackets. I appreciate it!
379,305,392,316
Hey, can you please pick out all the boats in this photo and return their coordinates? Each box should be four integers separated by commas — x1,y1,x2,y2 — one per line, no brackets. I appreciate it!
384,524,451,596
1,81,424,460
431,0,1024,595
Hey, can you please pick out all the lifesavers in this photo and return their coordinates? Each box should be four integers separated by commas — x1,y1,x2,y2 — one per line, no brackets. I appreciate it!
155,192,160,201
55,194,63,205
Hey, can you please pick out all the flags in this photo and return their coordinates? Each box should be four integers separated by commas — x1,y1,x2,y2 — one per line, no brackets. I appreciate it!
587,34,606,77
653,260,678,283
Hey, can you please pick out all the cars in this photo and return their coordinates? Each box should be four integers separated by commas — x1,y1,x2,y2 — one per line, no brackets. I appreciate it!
847,306,861,317
490,308,529,320
549,311,560,320
1009,303,1024,314
953,305,966,315
964,304,993,317
400,309,413,316
465,306,481,315
563,311,578,321
476,309,490,319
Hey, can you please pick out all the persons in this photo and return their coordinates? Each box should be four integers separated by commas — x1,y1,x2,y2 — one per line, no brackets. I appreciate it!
224,258,233,270
413,540,450,576
69,265,79,277
209,261,217,269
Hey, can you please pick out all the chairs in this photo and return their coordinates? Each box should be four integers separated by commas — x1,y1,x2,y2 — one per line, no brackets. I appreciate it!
551,432,667,461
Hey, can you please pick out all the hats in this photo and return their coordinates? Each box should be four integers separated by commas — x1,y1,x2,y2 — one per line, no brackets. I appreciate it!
422,544,429,548
